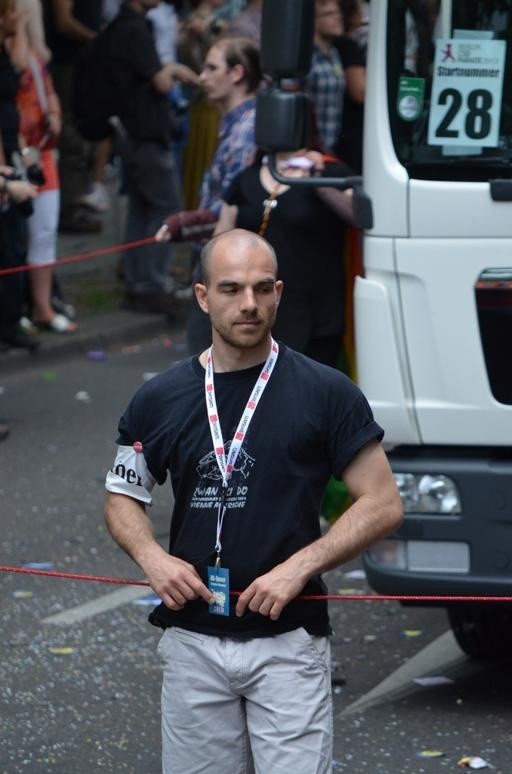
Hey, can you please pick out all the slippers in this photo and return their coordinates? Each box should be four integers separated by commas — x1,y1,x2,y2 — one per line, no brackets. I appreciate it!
39,313,81,334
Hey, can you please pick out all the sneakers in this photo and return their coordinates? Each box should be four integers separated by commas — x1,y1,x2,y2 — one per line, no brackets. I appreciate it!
58,182,110,233
4,326,42,351
53,295,77,320
118,287,179,313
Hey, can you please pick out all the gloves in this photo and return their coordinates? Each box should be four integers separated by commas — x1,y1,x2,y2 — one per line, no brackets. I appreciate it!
164,208,213,246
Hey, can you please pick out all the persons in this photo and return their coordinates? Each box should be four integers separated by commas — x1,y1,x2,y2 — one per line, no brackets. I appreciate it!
156,40,374,369
100,226,408,774
105,3,263,313
1,1,111,349
306,1,368,176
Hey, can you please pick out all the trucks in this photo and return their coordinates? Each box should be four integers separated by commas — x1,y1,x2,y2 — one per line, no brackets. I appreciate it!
256,0,512,661
